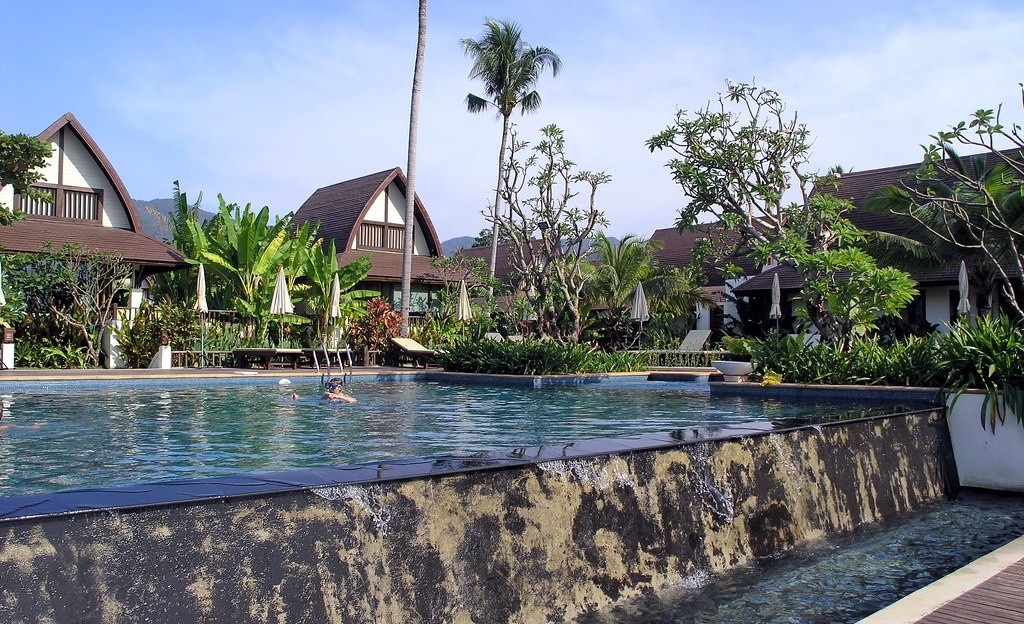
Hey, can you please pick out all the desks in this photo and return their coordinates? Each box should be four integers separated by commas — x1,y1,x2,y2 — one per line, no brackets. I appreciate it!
232,347,304,371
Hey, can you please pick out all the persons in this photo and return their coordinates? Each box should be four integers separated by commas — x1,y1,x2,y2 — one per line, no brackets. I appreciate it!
293,378,356,402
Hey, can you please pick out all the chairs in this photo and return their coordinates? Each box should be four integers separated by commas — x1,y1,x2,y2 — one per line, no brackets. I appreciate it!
391,337,450,369
624,329,714,354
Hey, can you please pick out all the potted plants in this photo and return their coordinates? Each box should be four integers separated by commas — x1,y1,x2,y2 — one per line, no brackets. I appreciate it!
930,313,1024,491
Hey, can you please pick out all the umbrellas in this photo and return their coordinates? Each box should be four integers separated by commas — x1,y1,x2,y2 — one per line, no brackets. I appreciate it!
270,267,292,367
456,280,472,341
957,261,970,316
330,273,341,366
194,263,208,367
630,282,649,329
769,274,782,339
